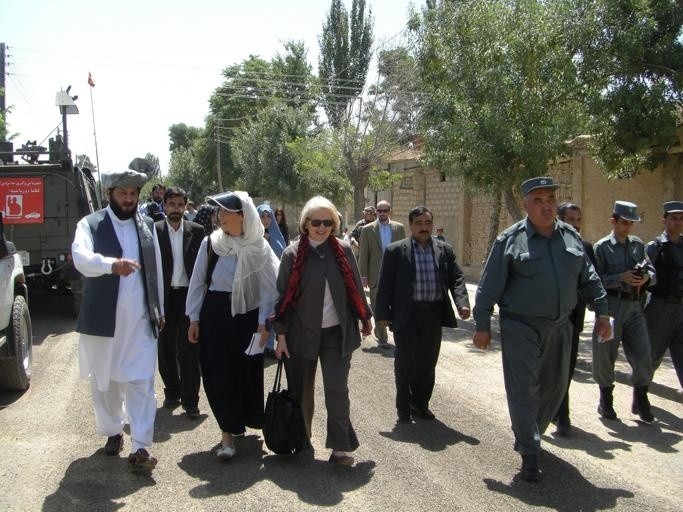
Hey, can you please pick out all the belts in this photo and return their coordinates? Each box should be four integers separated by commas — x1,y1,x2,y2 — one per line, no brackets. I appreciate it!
605,289,641,302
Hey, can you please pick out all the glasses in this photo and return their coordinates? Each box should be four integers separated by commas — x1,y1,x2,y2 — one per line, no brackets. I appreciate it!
377,210,390,213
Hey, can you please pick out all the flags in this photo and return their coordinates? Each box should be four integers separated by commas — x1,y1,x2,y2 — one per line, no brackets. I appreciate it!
87,72,96,88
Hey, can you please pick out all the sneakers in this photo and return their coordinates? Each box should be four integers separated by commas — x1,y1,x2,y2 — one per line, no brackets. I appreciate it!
104,396,246,474
521,456,543,481
378,342,394,349
329,455,355,467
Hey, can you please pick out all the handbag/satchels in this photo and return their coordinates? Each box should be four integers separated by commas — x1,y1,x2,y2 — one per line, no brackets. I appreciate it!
262,393,310,455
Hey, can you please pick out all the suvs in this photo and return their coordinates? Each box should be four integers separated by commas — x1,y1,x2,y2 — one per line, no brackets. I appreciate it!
1,209,36,395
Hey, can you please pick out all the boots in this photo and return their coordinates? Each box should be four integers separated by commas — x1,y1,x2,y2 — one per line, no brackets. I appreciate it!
598,385,617,419
631,385,654,422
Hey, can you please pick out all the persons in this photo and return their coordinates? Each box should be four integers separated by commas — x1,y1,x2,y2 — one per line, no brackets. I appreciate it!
372,203,474,425
273,192,377,470
69,165,169,480
154,185,208,422
181,187,281,462
591,199,658,426
347,199,407,346
552,199,604,440
139,183,220,238
432,226,446,242
645,199,683,393
470,173,617,485
256,204,290,258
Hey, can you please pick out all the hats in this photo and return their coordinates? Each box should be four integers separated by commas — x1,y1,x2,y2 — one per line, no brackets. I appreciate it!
521,177,560,197
663,201,683,215
305,217,334,227
613,200,641,222
204,190,247,212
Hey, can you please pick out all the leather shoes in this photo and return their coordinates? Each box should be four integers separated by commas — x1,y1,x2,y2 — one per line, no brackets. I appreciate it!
412,409,435,419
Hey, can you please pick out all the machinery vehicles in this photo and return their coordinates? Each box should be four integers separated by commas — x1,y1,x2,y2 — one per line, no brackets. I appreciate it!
1,132,101,316
